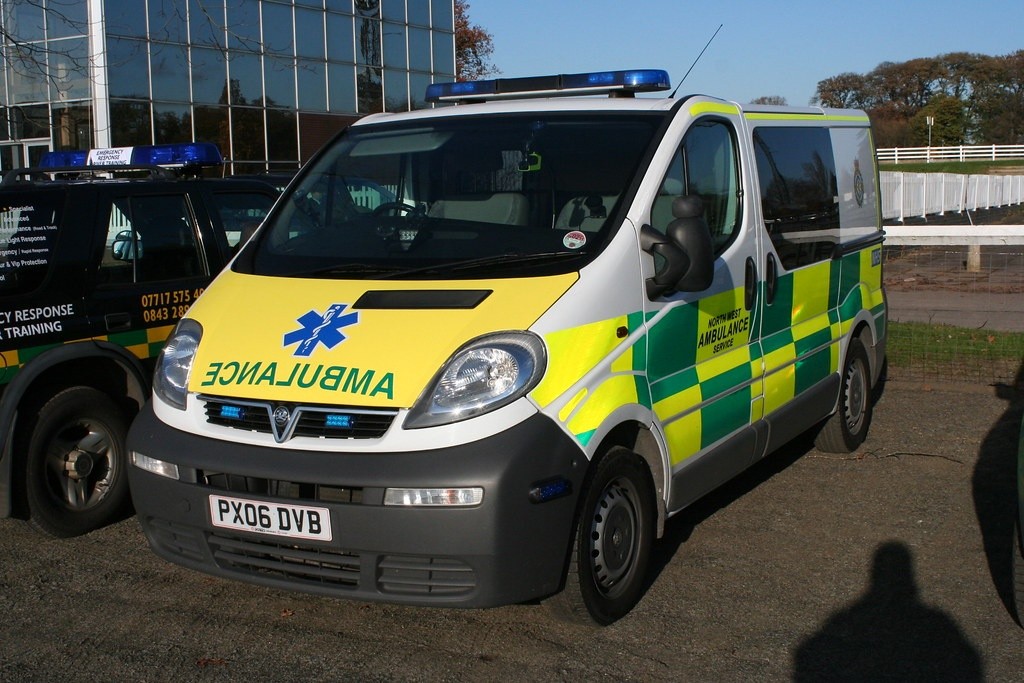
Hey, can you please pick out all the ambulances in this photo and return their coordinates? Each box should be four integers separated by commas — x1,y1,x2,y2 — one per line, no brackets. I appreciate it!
125,69,888,629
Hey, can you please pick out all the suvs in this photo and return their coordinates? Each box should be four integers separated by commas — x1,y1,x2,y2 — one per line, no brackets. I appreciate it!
0,145,281,535
219,170,399,249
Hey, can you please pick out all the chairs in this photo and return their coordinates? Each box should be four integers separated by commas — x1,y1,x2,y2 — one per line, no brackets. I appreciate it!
429,193,530,226
555,193,707,235
